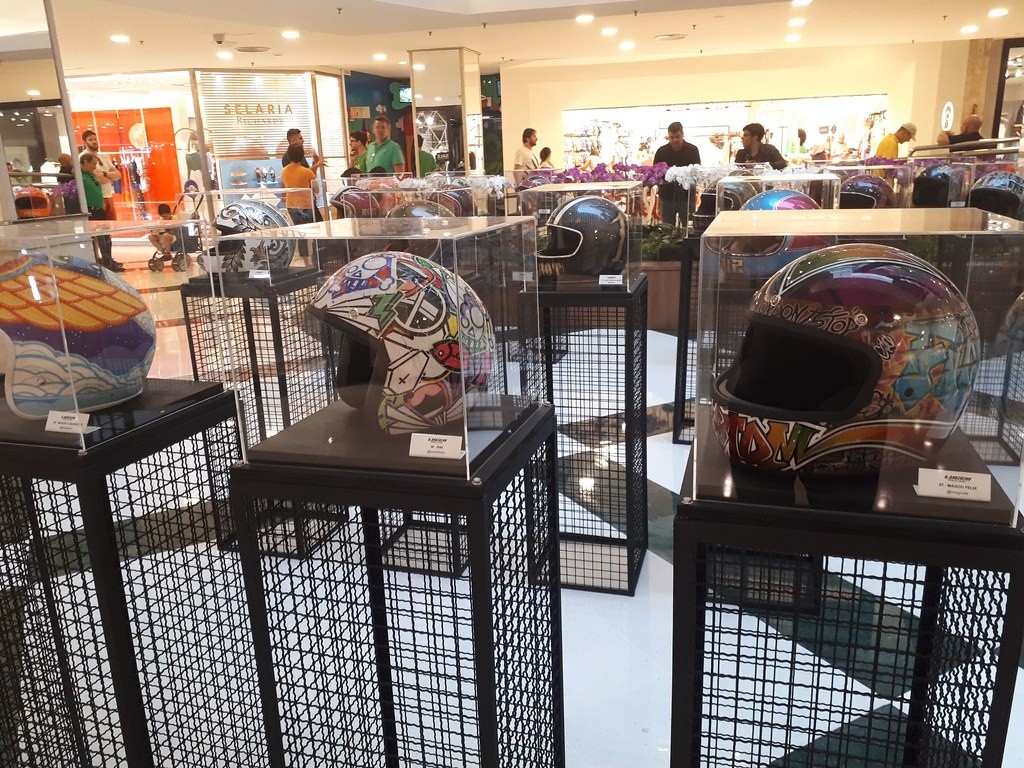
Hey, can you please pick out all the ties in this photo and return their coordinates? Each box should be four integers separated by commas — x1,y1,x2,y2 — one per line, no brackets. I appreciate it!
97,157,103,166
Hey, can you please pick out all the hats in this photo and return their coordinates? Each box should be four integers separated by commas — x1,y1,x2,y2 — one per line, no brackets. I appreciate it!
902,123,917,141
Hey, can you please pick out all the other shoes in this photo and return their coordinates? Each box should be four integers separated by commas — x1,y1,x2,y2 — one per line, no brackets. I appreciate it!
159,255,172,260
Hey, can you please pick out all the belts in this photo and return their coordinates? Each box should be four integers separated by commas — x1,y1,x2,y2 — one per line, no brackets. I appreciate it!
88,206,102,211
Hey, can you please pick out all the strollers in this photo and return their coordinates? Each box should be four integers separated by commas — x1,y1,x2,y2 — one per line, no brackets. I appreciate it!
149,188,205,272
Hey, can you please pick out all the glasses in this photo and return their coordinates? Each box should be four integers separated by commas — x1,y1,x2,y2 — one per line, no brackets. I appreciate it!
350,140,357,143
90,176,99,186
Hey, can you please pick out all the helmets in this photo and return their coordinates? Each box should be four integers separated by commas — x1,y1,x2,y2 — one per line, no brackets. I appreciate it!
430,184,478,216
531,195,629,276
329,186,379,218
384,200,456,270
710,243,980,477
718,189,828,278
307,251,496,440
0,248,157,420
840,175,895,208
912,164,962,208
965,171,1024,261
515,175,559,219
692,177,757,230
197,199,296,273
728,170,762,194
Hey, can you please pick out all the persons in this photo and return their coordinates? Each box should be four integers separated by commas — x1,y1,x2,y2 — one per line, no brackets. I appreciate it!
349,131,367,174
56,130,128,272
873,123,917,189
365,115,406,177
280,128,328,266
936,113,992,159
653,121,701,227
7,161,29,183
734,123,787,169
512,127,555,212
417,134,436,178
147,204,180,259
